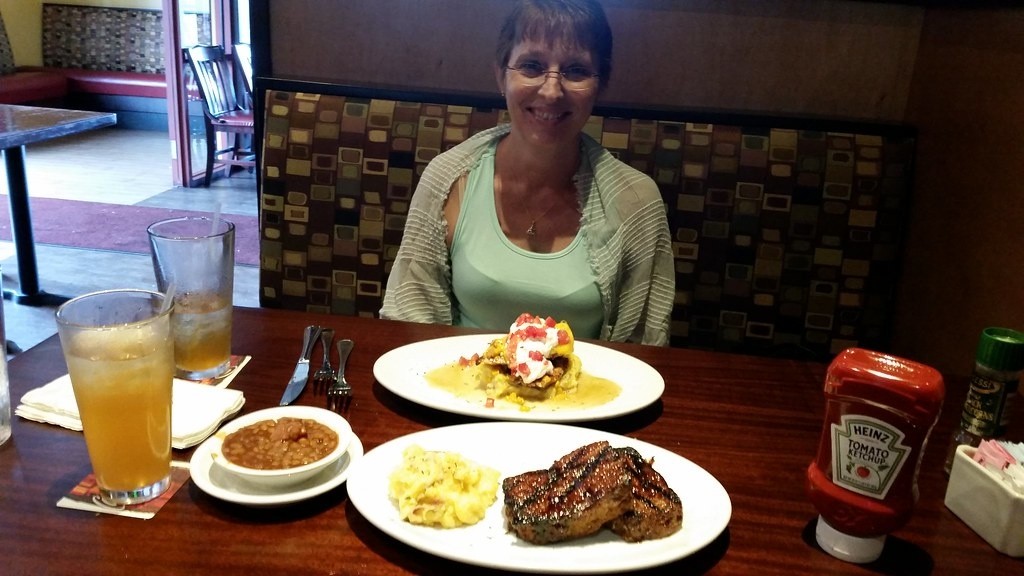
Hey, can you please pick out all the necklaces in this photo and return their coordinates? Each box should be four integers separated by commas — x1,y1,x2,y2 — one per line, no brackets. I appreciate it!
506,153,580,239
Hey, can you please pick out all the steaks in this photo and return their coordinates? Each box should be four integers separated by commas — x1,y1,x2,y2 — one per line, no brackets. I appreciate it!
501,438,632,544
598,446,683,543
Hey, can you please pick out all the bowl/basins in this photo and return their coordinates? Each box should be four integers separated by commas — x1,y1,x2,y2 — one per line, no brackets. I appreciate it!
211,405,353,486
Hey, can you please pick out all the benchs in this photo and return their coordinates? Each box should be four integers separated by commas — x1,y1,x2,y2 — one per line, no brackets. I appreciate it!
252,74,909,367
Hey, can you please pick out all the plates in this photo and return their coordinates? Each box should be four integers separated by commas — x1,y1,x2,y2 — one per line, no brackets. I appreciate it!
346,421,732,575
372,334,666,423
189,432,364,508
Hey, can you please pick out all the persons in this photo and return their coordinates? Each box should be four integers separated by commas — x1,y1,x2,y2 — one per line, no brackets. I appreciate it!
379,0,675,347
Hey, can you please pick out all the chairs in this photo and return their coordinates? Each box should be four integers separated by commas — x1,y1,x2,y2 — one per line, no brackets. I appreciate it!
188,44,254,188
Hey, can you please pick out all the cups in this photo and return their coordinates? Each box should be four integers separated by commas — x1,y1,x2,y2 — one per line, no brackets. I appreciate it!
0,264,12,446
147,215,235,380
55,287,175,505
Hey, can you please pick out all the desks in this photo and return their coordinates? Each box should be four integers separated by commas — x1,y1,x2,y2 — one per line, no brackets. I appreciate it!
0,105,117,292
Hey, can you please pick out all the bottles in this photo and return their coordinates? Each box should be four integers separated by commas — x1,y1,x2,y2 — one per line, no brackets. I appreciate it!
946,327,1024,476
804,347,944,565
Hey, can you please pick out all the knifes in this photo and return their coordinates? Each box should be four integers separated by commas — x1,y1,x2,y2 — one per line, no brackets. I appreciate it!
280,326,321,405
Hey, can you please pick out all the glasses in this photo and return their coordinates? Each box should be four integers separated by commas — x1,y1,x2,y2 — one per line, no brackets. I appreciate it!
504,61,601,91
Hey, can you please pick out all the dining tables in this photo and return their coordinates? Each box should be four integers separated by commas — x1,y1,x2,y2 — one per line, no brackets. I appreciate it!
0,307,1024,576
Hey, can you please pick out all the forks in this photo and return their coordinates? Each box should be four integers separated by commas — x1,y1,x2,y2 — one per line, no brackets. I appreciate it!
312,327,338,397
327,339,354,415
